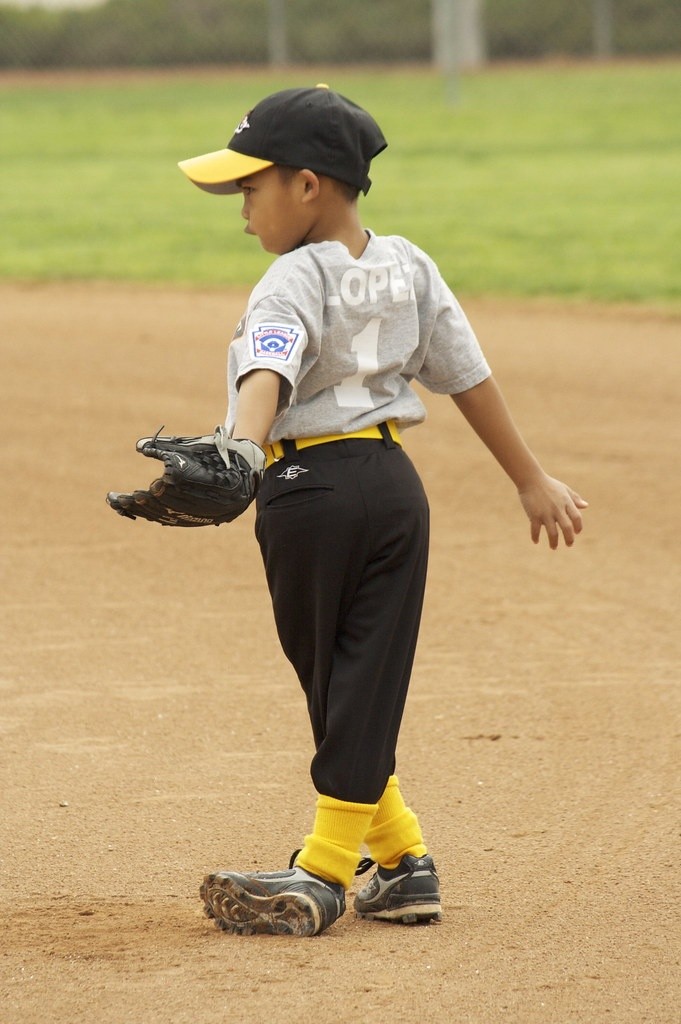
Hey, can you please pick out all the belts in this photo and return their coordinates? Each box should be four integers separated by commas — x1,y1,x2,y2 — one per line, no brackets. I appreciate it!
261,417,404,473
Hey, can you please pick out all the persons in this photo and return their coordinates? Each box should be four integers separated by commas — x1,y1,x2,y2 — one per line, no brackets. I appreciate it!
176,81,590,937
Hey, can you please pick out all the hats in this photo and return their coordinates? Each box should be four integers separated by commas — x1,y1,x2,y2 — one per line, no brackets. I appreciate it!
175,84,388,197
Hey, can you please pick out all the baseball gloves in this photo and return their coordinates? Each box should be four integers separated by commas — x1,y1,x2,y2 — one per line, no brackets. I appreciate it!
104,422,269,532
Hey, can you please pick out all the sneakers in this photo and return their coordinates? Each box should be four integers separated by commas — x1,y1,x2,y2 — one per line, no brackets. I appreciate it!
198,848,348,936
352,853,444,925
